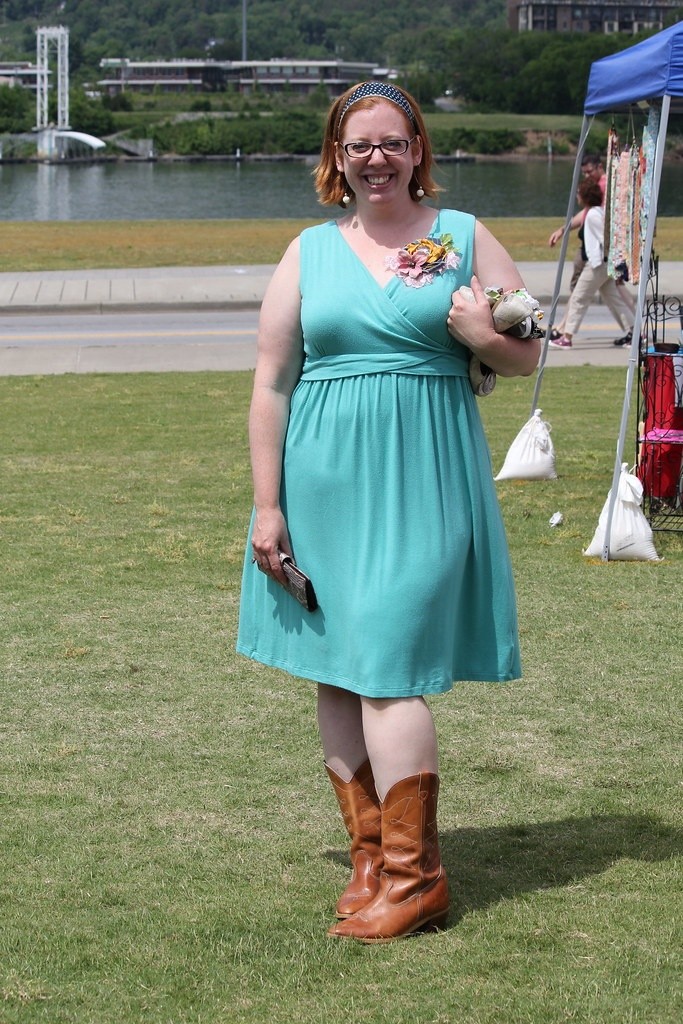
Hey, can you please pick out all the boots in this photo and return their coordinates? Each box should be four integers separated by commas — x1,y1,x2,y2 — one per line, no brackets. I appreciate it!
322,758,384,919
326,772,451,944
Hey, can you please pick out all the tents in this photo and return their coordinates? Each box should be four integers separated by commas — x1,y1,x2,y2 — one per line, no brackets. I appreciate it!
533,22,683,561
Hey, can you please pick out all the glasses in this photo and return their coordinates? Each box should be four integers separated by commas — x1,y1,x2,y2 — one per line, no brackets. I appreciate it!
582,163,597,174
338,136,415,159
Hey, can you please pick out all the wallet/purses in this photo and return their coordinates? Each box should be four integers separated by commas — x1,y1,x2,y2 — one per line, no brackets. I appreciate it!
251,549,318,612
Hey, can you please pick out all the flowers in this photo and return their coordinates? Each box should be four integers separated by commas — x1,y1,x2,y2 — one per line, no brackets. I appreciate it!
385,232,462,288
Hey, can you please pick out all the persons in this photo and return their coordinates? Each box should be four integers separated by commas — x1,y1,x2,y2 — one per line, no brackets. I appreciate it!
233,82,543,944
542,152,648,351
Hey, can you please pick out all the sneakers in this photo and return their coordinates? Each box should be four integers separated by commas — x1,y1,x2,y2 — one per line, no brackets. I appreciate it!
541,327,561,341
622,334,644,348
614,332,641,345
548,336,572,349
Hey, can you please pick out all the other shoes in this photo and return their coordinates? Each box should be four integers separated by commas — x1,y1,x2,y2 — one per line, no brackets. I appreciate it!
469,291,533,398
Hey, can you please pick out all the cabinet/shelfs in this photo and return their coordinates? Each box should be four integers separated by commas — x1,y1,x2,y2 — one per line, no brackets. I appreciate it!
640,243,683,534
